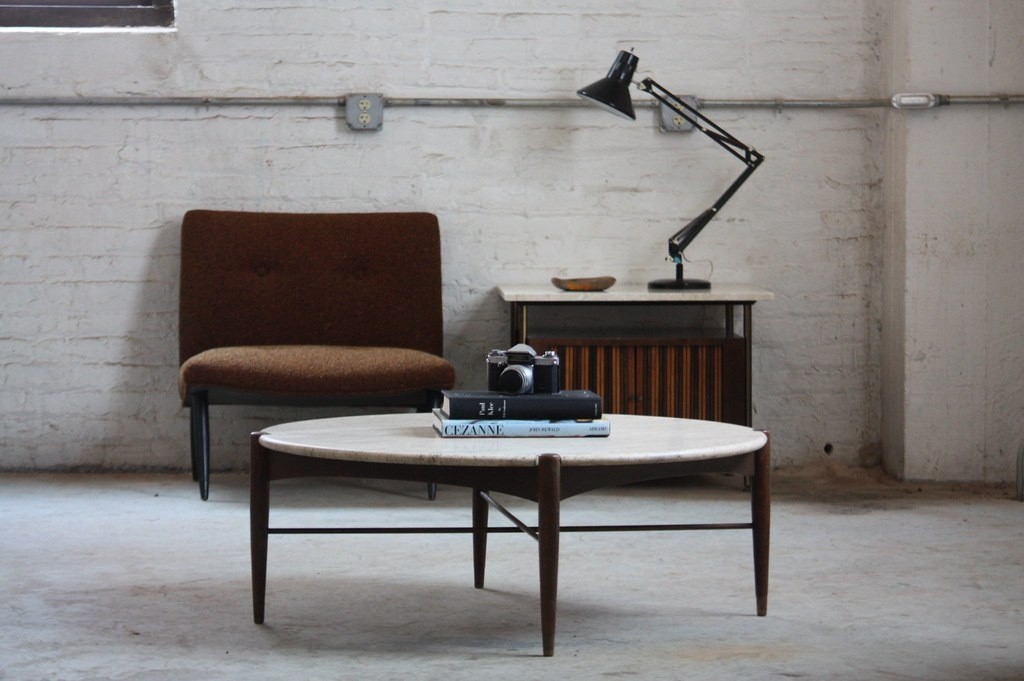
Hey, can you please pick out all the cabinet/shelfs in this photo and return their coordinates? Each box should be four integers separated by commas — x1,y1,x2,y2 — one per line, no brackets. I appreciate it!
526,327,744,475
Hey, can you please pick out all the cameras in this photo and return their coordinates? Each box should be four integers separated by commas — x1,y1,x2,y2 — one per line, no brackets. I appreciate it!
486,342,561,396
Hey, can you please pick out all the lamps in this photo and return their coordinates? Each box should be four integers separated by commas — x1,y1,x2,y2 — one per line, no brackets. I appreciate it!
576,47,765,293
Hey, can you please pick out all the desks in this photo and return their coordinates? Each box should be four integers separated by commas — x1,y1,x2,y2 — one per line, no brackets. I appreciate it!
250,413,772,657
496,282,773,492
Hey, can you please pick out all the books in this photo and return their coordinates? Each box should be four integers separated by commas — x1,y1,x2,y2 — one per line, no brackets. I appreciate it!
431,408,611,438
441,389,602,420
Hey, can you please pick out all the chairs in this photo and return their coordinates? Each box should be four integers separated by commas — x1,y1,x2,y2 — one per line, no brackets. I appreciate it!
180,209,454,502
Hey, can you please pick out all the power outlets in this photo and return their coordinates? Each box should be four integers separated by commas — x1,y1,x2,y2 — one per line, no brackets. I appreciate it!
345,94,384,130
660,95,700,131
